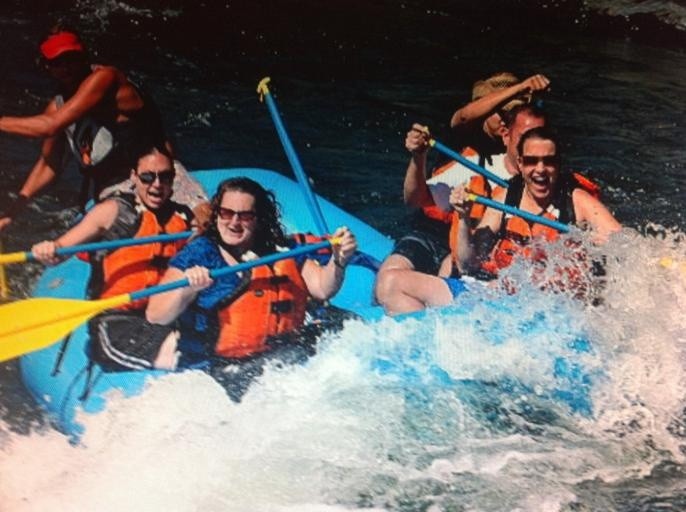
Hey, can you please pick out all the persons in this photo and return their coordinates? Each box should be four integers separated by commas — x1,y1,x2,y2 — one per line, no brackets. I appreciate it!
374,69,554,276
1,32,167,238
146,177,360,408
375,125,624,315
401,103,550,279
29,143,205,376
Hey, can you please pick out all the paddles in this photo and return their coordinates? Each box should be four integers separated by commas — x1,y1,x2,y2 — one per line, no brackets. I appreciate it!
466,194,686,276
0,236,349,369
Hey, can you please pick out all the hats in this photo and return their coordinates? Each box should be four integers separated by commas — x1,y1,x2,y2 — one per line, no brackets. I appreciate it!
41,31,88,61
473,70,533,113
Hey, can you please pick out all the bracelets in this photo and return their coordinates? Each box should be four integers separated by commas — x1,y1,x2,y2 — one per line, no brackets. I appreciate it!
331,256,346,272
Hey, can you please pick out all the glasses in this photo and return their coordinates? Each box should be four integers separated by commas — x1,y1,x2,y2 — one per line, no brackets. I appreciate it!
214,207,259,223
520,155,558,167
137,170,176,187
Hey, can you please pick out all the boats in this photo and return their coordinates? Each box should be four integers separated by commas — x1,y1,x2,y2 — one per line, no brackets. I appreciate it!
421,236,655,407
16,164,401,462
